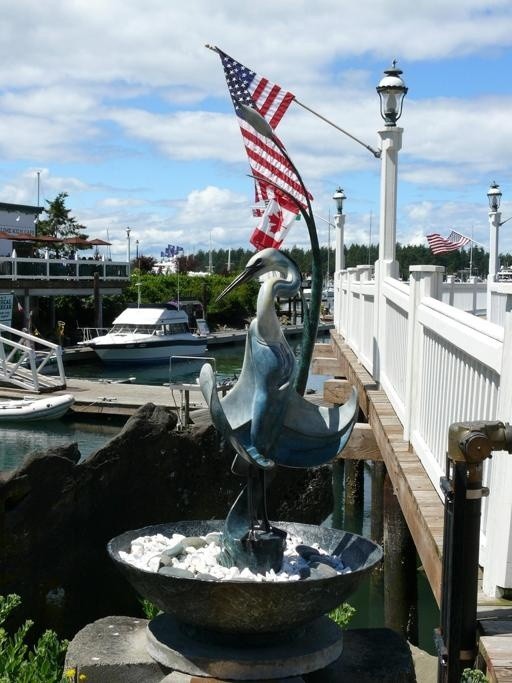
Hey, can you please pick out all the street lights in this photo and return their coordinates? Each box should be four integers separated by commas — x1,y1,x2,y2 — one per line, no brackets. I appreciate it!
331,184,347,270
375,54,407,259
125,225,131,261
486,179,502,275
135,239,140,261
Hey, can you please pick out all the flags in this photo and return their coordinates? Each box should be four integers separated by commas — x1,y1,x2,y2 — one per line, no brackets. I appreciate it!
216,44,314,222
425,229,469,254
249,196,302,252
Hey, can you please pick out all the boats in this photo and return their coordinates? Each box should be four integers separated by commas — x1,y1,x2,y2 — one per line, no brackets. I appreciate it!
0,393,76,426
78,259,209,364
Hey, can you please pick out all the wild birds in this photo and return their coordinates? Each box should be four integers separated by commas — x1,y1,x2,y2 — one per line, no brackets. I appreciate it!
197,247,360,540
